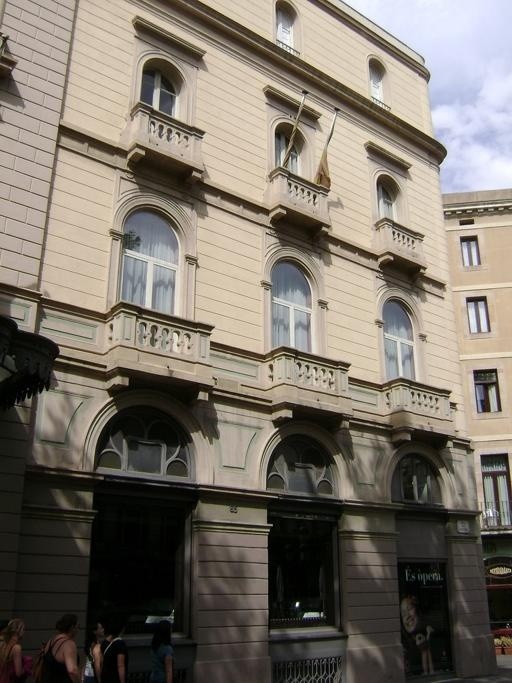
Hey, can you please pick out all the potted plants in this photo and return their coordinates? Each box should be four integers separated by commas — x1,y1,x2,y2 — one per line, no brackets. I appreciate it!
493,637,503,655
502,636,512,656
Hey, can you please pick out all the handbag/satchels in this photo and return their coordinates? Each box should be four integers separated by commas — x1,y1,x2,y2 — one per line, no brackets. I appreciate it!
31,639,49,683
0,643,17,683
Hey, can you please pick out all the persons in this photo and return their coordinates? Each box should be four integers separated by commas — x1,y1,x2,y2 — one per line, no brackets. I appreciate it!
99,620,129,683
149,627,176,683
401,597,447,676
0,618,25,683
83,622,105,683
0,620,9,646
41,614,82,683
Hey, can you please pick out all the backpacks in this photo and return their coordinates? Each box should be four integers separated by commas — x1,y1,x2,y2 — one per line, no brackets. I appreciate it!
80,656,95,683
